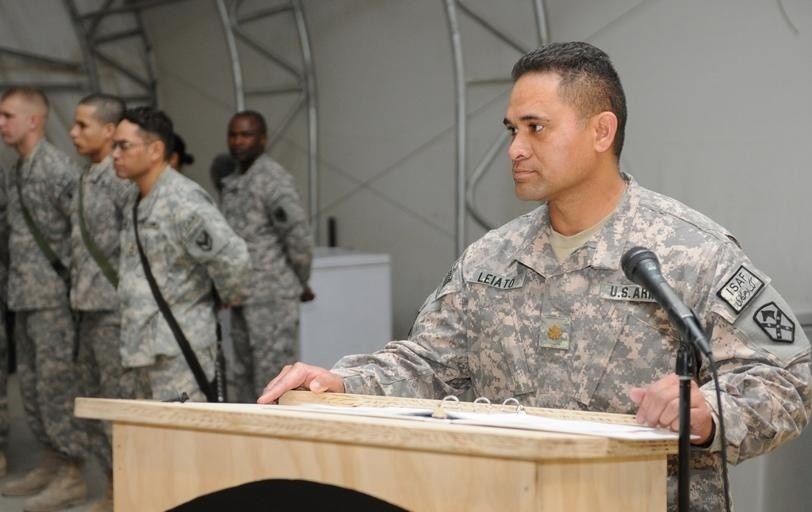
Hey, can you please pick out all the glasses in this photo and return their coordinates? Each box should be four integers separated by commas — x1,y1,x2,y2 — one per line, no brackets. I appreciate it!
110,141,153,151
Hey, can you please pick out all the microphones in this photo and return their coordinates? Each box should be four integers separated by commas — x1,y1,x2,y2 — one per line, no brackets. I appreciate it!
621,247,712,357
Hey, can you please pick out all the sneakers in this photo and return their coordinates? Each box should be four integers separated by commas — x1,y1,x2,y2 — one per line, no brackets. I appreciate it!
0,462,86,512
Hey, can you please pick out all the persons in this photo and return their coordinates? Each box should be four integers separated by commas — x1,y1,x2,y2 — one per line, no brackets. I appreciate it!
0,85,89,512
110,105,260,401
218,108,315,402
255,41,812,512
68,91,137,512
169,133,194,172
0,166,14,477
